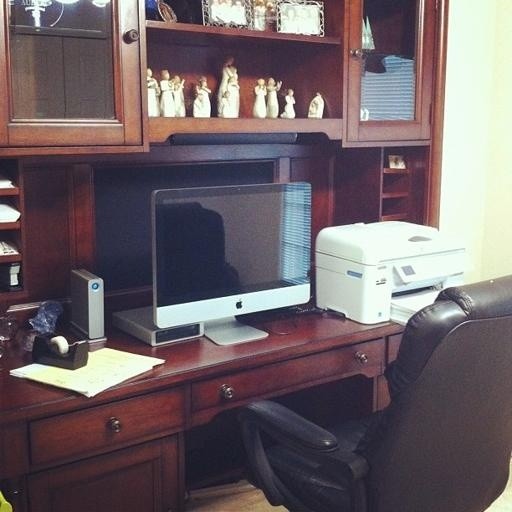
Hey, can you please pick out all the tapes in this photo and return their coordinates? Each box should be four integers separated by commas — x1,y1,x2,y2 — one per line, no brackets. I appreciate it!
50,336,69,354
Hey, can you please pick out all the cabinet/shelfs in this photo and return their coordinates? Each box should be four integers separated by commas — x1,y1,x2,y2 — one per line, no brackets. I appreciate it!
0,0,150,157
345,150,410,222
146,19,343,143
292,0,449,149
0,157,28,301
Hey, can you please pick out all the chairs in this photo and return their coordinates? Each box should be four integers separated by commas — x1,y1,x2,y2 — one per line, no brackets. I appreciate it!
208,275,512,512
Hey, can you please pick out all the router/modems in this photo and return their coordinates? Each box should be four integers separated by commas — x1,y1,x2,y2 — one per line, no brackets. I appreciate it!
112,306,205,346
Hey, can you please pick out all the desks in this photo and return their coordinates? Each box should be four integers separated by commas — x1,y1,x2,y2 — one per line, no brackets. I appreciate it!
0,297,403,512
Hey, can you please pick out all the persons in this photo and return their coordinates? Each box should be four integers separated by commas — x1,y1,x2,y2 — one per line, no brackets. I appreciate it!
254,0,277,31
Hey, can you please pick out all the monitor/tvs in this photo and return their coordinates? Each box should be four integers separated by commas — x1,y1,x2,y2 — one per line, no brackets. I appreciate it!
151,181,313,345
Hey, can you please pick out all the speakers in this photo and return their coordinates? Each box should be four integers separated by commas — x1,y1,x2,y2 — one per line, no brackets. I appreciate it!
71,268,105,340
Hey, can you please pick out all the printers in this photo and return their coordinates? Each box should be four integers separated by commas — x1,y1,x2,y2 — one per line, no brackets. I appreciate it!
315,220,468,326
